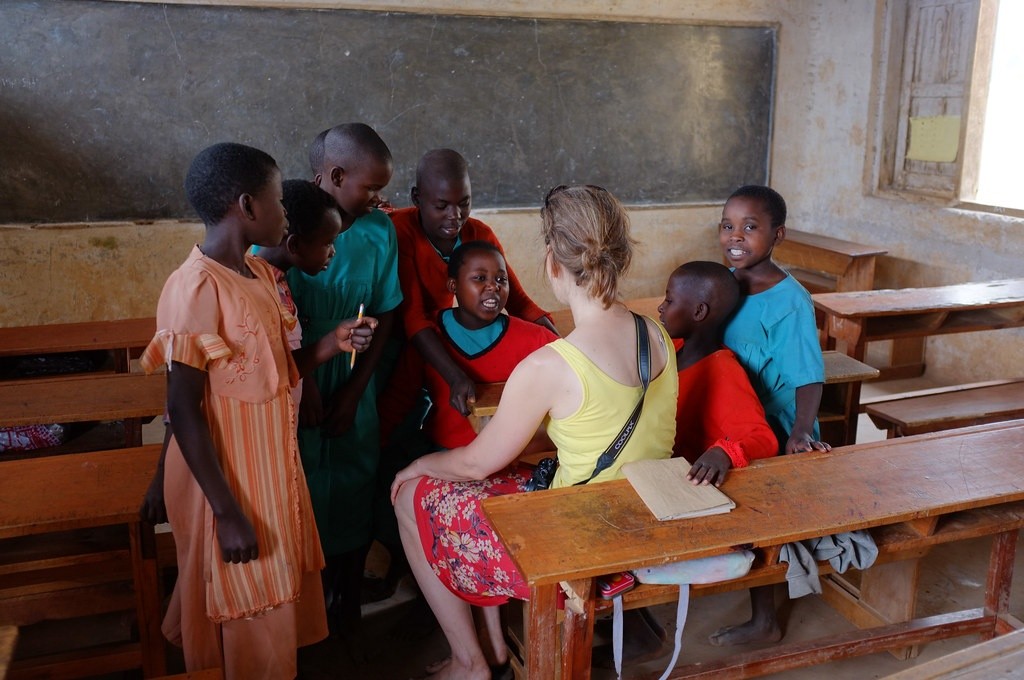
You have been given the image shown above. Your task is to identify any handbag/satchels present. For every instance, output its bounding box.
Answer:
[632,550,755,584]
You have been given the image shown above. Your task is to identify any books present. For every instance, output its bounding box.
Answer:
[622,457,736,521]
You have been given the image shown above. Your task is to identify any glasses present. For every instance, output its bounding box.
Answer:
[545,184,607,208]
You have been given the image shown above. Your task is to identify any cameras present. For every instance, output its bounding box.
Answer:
[525,456,557,492]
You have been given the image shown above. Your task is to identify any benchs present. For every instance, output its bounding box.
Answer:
[865,379,1023,440]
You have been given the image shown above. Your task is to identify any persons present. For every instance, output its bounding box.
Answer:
[591,261,779,669]
[134,123,565,680]
[389,185,679,680]
[710,185,831,647]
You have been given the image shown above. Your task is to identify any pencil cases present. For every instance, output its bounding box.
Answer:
[596,572,635,601]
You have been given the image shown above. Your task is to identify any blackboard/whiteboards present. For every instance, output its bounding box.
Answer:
[0,0,781,226]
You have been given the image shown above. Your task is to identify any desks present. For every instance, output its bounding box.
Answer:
[1,226,1022,680]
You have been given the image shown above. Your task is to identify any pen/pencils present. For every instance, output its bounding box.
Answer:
[351,304,364,370]
